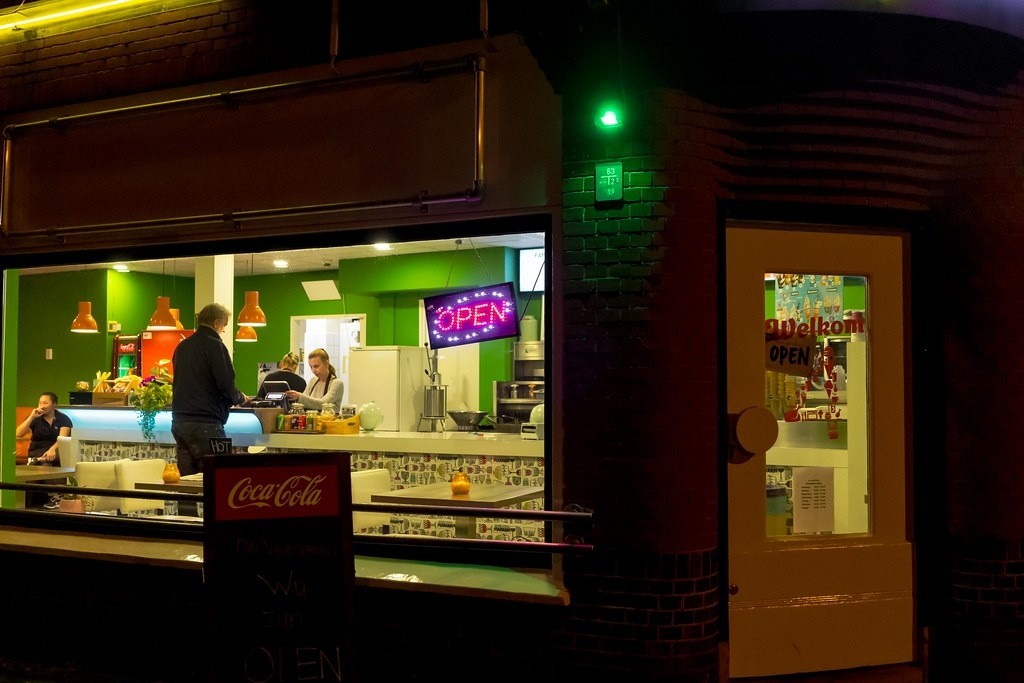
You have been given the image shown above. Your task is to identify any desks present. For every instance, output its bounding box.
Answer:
[15,464,76,508]
[371,480,545,539]
[134,473,203,493]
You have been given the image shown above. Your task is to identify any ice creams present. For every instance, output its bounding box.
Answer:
[777,275,840,323]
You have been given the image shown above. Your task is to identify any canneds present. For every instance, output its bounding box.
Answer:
[278,414,314,430]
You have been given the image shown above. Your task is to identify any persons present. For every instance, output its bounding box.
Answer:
[285,348,344,415]
[257,352,307,415]
[171,303,249,517]
[16,392,73,509]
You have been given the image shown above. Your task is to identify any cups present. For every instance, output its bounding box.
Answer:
[93,379,104,392]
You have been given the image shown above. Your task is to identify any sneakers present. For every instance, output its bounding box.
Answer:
[27,457,41,466]
[43,498,61,509]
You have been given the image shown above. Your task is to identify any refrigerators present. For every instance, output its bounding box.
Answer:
[348,345,430,432]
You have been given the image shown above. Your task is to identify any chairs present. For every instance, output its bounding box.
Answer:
[74,455,203,522]
[351,469,391,534]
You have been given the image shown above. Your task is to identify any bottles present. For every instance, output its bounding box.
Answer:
[277,403,355,430]
[510,384,519,399]
[128,388,134,406]
[452,466,470,494]
[162,455,180,483]
[528,384,537,399]
[519,315,538,342]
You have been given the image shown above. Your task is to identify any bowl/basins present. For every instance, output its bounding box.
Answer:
[447,410,488,431]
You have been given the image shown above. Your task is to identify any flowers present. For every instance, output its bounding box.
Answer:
[130,376,173,450]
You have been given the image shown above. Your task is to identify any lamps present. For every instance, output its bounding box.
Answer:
[71,263,99,333]
[149,258,184,330]
[236,254,267,342]
